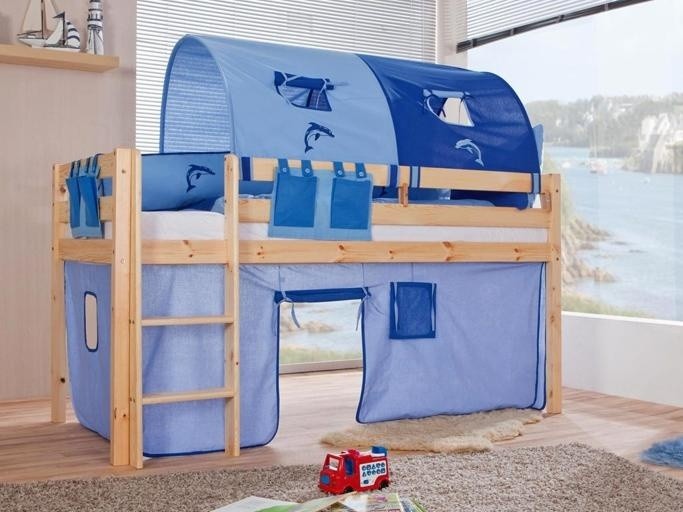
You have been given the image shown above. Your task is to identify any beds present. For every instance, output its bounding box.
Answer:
[47,146,564,472]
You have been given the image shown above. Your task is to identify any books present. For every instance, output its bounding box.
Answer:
[209,490,425,511]
